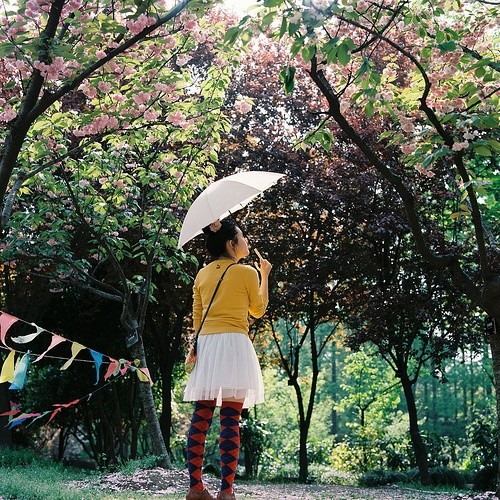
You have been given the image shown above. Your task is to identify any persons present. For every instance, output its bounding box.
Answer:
[181,219,272,500]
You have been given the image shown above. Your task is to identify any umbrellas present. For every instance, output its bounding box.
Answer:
[177,171,288,273]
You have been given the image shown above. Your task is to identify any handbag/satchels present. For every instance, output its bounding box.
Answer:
[185,340,198,373]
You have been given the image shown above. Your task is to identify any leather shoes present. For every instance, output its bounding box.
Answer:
[186,488,217,500]
[217,491,237,500]
